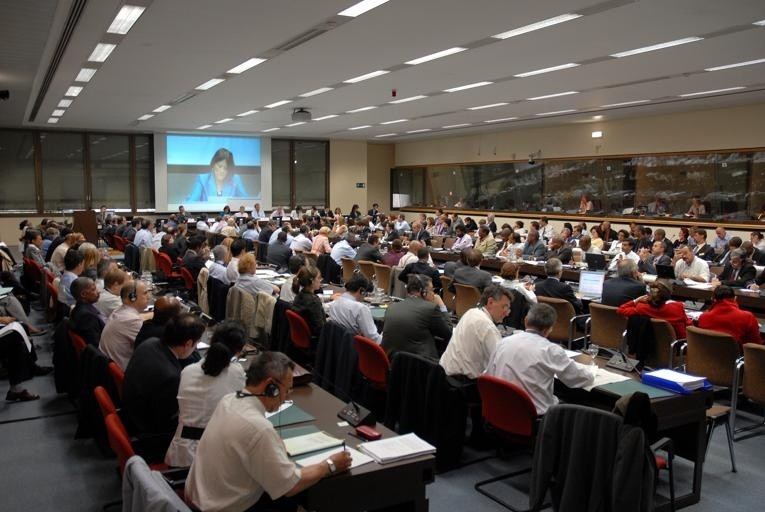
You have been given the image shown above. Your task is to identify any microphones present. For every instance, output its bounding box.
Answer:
[496,320,516,336]
[597,343,640,372]
[673,285,705,312]
[522,264,537,283]
[305,364,376,428]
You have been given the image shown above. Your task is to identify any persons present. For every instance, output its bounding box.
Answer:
[577,193,594,215]
[1,201,764,511]
[686,195,706,218]
[183,146,248,202]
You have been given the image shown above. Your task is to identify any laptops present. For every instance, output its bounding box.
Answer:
[575,270,605,300]
[585,253,608,270]
[656,264,676,280]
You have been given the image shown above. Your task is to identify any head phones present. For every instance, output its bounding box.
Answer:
[414,274,427,297]
[129,279,138,301]
[353,269,369,296]
[236,384,280,398]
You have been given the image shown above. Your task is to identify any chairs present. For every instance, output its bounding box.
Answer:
[0,213,765,511]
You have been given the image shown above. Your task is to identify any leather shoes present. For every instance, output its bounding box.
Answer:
[30,329,47,336]
[35,366,53,376]
[6,389,40,401]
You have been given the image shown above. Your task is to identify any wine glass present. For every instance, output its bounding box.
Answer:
[589,344,599,366]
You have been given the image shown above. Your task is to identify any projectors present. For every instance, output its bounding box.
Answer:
[292,108,311,121]
